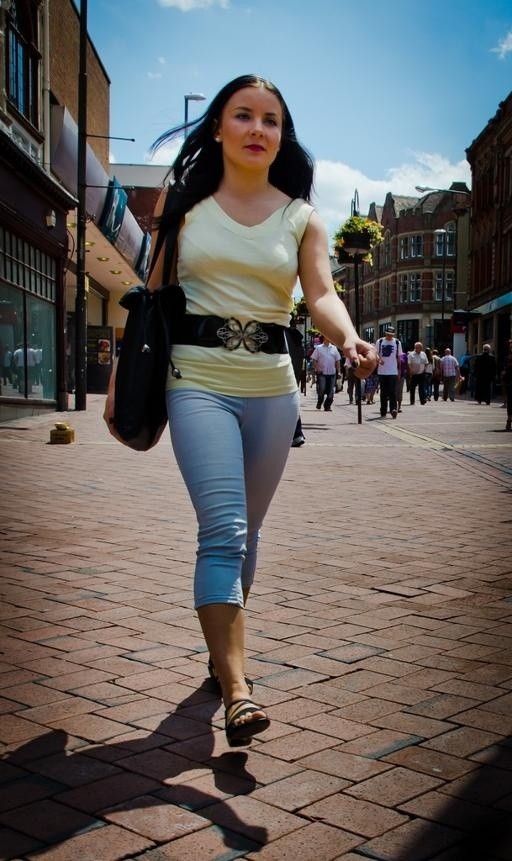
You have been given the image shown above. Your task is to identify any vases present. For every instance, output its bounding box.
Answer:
[308,331,319,338]
[338,292,344,300]
[297,303,308,313]
[337,251,363,264]
[342,232,370,248]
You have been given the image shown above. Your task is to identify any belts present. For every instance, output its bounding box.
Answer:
[172,315,292,354]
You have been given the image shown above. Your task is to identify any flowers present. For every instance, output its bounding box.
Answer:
[334,281,346,293]
[335,215,385,247]
[333,243,374,268]
[305,326,320,336]
[297,299,306,304]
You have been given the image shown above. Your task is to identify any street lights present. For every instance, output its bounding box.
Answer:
[415,186,475,358]
[432,225,448,347]
[184,92,205,176]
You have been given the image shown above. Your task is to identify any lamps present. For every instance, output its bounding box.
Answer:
[45,208,57,229]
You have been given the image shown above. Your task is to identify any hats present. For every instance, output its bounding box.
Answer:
[385,327,395,335]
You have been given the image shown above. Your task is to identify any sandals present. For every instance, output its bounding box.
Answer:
[226,700,270,747]
[207,656,252,698]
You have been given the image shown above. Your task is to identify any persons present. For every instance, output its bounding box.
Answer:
[1,340,44,395]
[101,72,382,749]
[298,322,512,437]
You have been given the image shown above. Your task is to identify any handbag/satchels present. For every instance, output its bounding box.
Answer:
[109,184,186,451]
